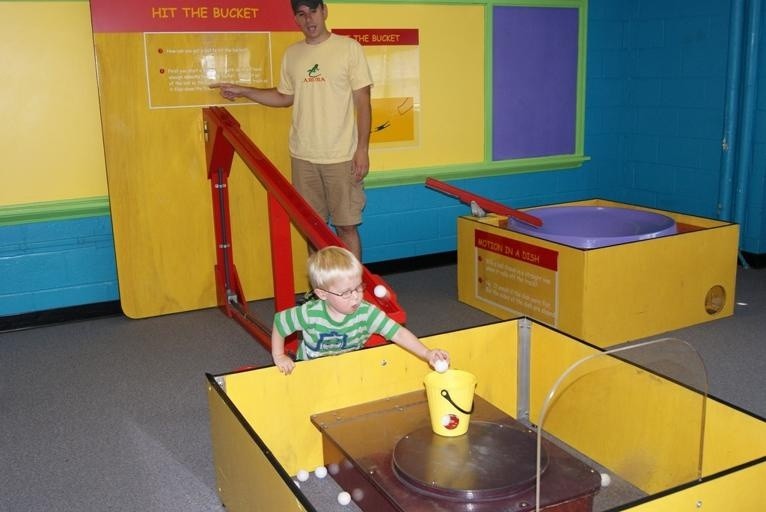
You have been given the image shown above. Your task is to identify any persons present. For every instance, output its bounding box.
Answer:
[265,248,451,382]
[209,1,372,305]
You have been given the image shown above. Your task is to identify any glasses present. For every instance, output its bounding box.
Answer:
[320,282,367,299]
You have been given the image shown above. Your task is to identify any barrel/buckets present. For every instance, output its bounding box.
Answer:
[424,370,479,439]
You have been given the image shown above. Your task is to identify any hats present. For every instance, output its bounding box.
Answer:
[291,0,322,9]
